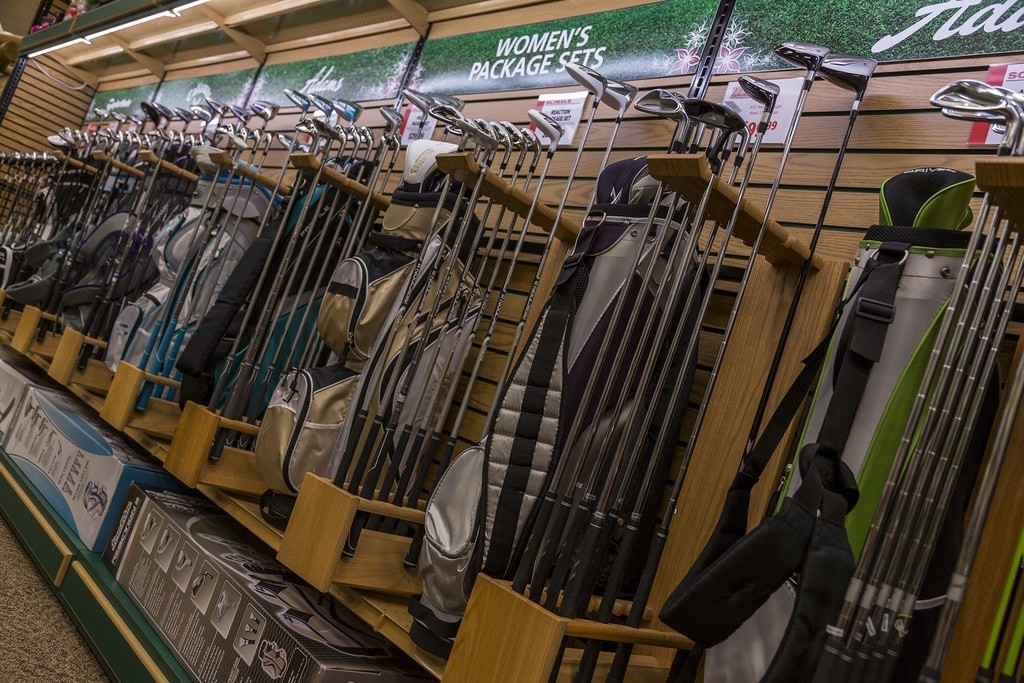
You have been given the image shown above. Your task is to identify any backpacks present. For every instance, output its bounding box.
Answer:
[318,244,417,360]
[149,206,211,282]
[254,364,360,496]
[105,284,171,374]
[405,431,490,658]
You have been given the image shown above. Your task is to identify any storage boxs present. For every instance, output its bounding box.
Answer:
[0,347,406,683]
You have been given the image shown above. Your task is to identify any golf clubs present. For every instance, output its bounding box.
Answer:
[813,78,1023,682]
[489,38,877,683]
[0,86,568,578]
